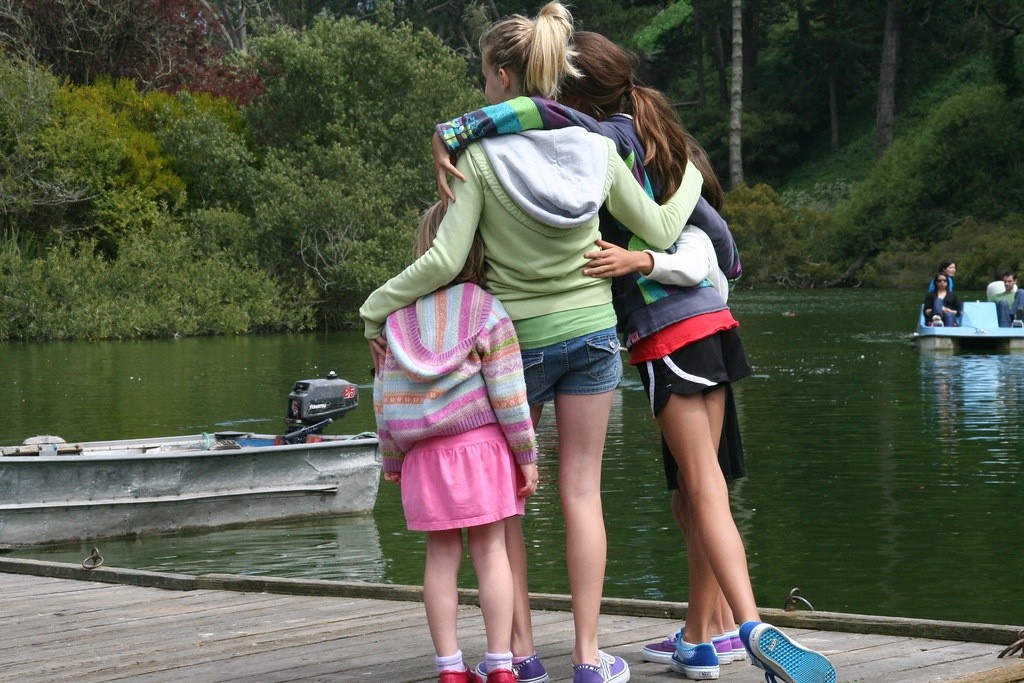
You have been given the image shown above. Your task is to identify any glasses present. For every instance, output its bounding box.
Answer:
[937,280,947,283]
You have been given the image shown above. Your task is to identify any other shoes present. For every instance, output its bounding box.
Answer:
[932,314,941,321]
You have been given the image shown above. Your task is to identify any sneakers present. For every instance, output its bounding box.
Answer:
[641,630,747,664]
[475,655,549,683]
[739,621,837,683]
[438,662,518,683]
[670,627,720,680]
[571,650,630,683]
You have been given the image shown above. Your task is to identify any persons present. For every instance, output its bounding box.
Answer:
[924,260,962,325]
[359,0,836,683]
[991,270,1024,328]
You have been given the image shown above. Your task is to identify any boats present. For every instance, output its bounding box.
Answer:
[911,281,1024,351]
[0,371,384,551]
